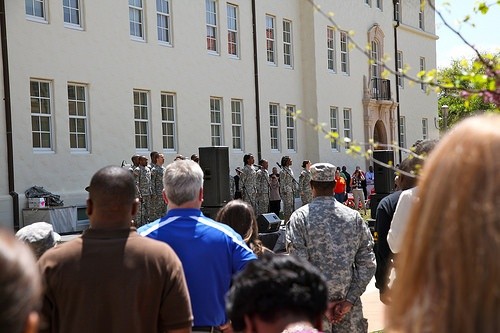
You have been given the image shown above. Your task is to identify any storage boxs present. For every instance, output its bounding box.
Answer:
[28,198,45,208]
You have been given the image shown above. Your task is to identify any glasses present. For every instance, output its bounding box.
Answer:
[84,180,139,193]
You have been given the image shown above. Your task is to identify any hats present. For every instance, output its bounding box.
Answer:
[14,222,62,255]
[306,162,337,185]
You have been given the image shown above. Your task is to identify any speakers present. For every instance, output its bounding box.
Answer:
[373,150,395,193]
[256,212,281,232]
[370,193,390,220]
[198,146,229,206]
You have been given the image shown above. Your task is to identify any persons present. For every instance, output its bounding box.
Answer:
[384,114,500,333]
[137,160,258,333]
[229,153,375,226]
[0,165,194,333]
[215,163,377,333]
[127,152,199,229]
[372,138,438,305]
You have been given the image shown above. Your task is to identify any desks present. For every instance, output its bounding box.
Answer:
[23,206,78,234]
